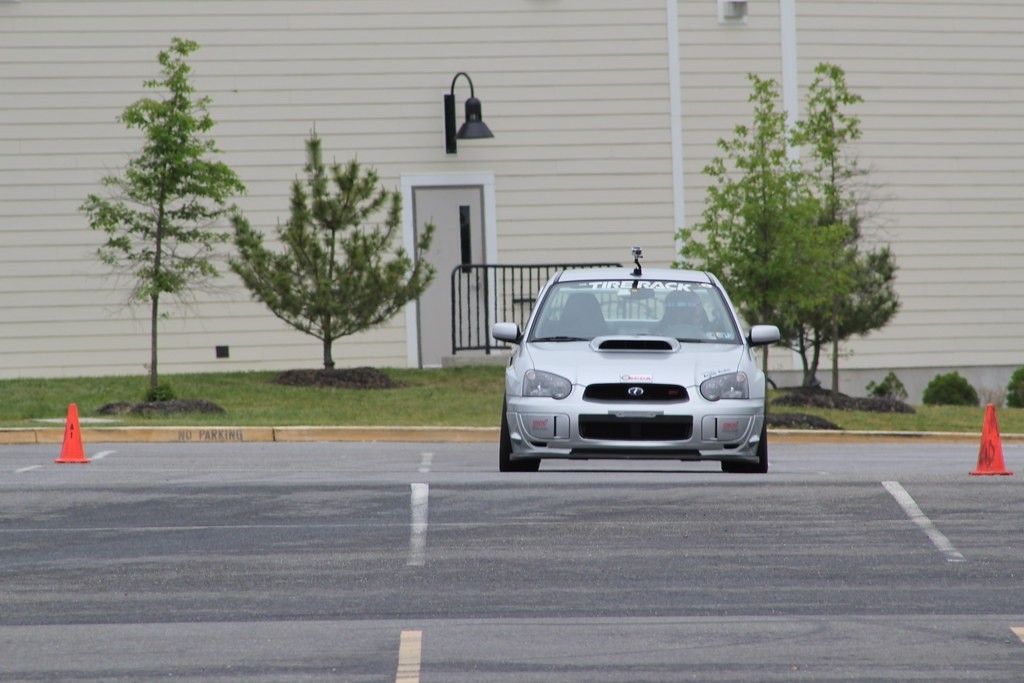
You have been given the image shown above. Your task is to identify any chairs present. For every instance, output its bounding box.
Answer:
[562,293,607,335]
[661,290,710,338]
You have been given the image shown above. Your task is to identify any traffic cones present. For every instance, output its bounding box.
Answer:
[55,401,90,465]
[967,402,1013,477]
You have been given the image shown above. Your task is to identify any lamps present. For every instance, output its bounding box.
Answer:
[444,73,494,153]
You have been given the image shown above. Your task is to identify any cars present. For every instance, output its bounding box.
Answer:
[492,263,781,473]
